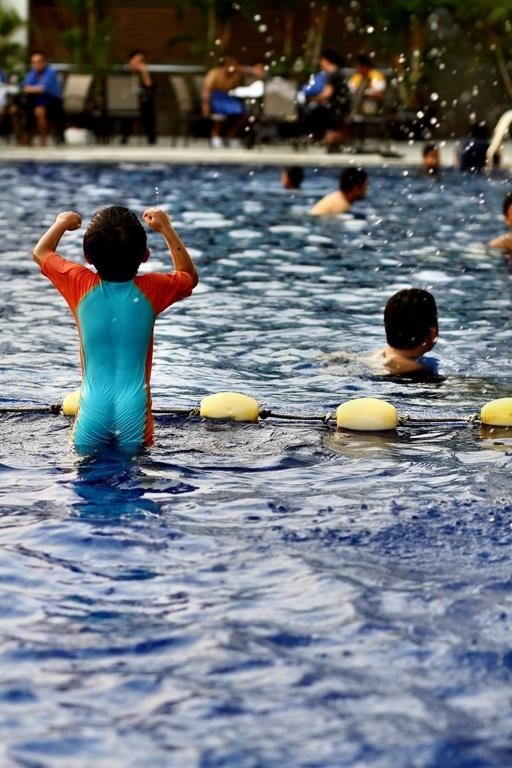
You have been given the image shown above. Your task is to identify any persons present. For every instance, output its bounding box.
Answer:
[201,46,387,148]
[281,166,306,189]
[7,49,64,147]
[0,68,9,120]
[486,190,512,267]
[32,204,198,451]
[356,286,440,381]
[413,140,441,180]
[310,166,370,216]
[117,46,158,143]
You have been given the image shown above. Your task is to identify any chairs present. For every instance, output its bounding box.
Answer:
[53,68,372,152]
